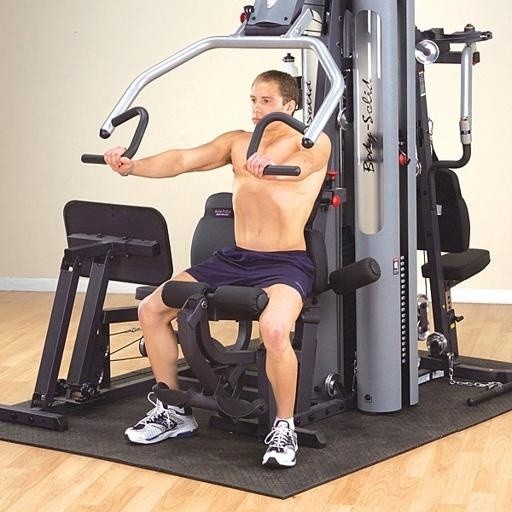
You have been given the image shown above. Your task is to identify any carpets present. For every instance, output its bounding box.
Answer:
[1,329,511,500]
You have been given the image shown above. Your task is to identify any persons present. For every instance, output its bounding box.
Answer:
[102,70,334,468]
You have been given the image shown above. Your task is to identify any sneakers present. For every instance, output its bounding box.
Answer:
[123,389,199,446]
[262,419,299,469]
